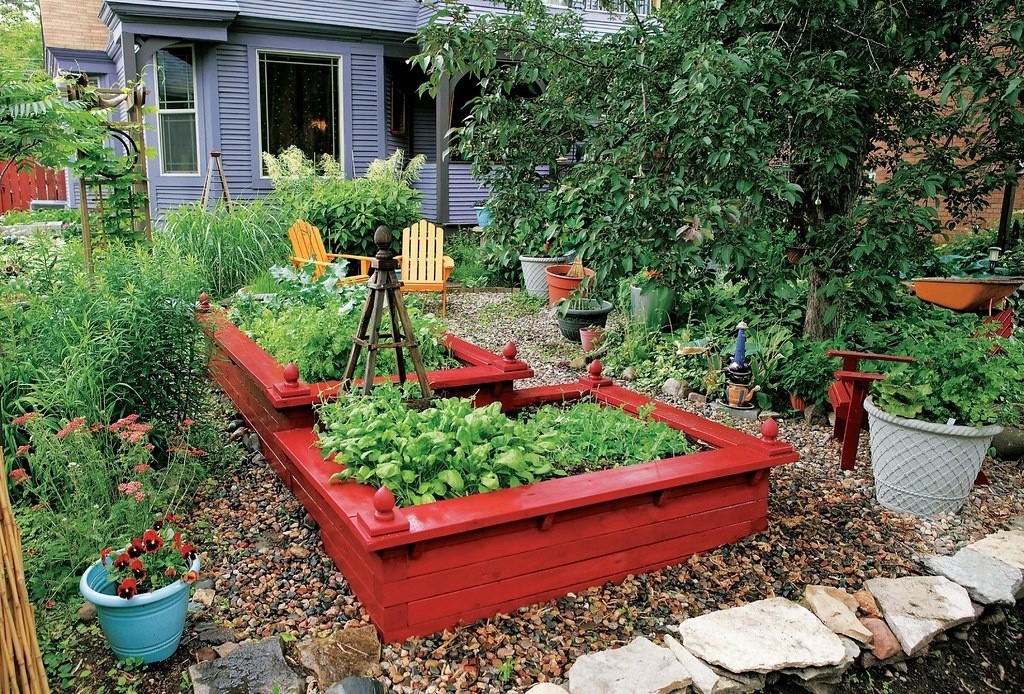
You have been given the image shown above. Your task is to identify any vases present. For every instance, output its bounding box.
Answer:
[630,284,654,324]
[545,264,596,308]
[80,548,200,665]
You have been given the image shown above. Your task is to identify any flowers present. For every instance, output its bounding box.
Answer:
[99,509,202,599]
[630,266,672,297]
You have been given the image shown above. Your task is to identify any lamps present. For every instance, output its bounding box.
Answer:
[988,246,1002,273]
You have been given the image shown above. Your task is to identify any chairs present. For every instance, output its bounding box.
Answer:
[392,220,454,317]
[826,308,1015,472]
[289,220,378,290]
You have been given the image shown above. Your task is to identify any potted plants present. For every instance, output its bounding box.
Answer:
[578,324,606,351]
[198,290,536,455]
[911,254,1024,310]
[862,318,1024,518]
[552,275,615,342]
[272,360,801,647]
[518,233,566,300]
